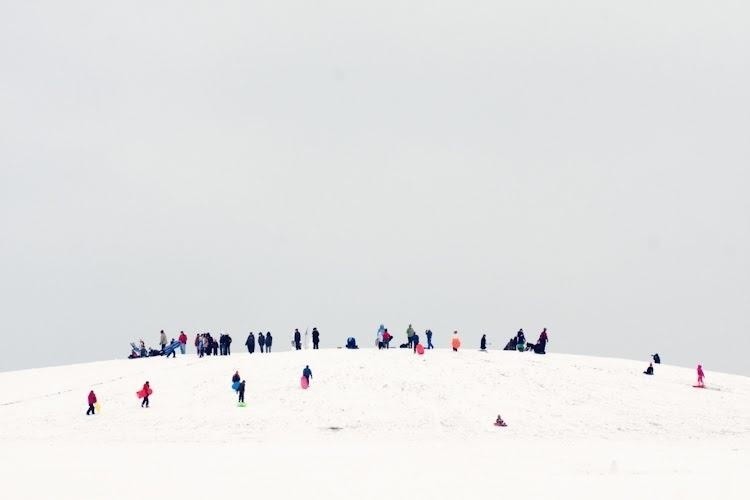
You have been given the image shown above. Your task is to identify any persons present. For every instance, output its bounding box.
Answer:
[481,335,487,350]
[653,354,660,364]
[232,370,240,394]
[239,380,245,403]
[697,365,704,386]
[245,332,255,354]
[415,342,424,354]
[166,338,177,358]
[408,332,419,354]
[496,414,507,426]
[407,326,414,348]
[87,391,97,415]
[346,336,358,349]
[258,332,266,353]
[294,329,302,350]
[383,329,390,349]
[452,330,460,351]
[312,328,320,349]
[266,332,272,353]
[303,365,312,387]
[517,338,525,352]
[130,345,161,358]
[195,332,219,358]
[160,329,167,350]
[644,364,654,375]
[517,329,524,342]
[179,331,187,354]
[425,330,434,350]
[378,332,384,349]
[219,333,231,355]
[141,381,151,408]
[538,328,548,350]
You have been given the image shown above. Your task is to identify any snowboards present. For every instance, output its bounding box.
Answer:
[301,377,307,388]
[137,388,153,398]
[494,420,508,427]
[452,337,461,348]
[239,400,246,407]
[93,403,101,412]
[163,342,181,357]
[417,344,423,353]
